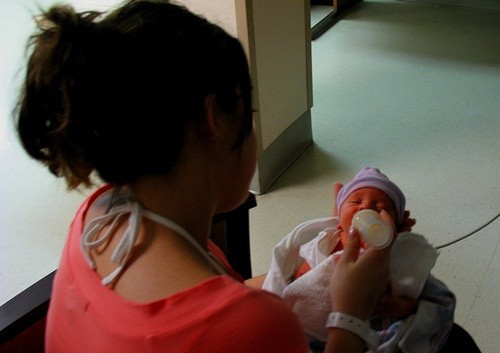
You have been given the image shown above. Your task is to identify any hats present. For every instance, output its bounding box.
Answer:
[337,166,407,221]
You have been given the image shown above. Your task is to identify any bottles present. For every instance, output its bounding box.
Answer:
[351,208,393,249]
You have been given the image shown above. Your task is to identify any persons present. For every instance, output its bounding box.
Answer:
[0,0,482,353]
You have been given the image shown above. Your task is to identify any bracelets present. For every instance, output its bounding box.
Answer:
[325,311,380,351]
